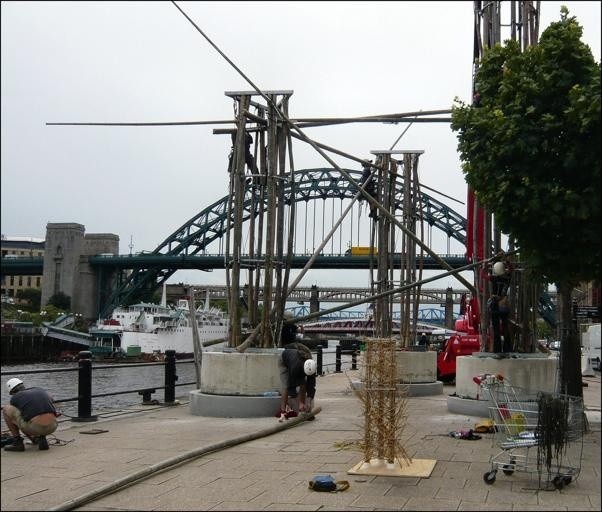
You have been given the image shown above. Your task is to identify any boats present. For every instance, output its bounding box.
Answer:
[93,302,229,360]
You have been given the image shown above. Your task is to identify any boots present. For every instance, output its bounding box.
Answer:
[38,435,49,450]
[4,437,24,451]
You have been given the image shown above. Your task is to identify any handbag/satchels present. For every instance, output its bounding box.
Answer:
[308,476,350,493]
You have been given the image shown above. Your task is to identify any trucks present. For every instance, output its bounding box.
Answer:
[345,247,378,257]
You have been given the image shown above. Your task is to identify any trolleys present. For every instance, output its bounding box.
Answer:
[471,371,586,489]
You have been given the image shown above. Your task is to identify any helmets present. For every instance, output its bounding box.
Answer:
[304,359,317,376]
[6,377,23,394]
[492,262,506,276]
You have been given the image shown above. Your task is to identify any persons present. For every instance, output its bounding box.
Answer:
[360,159,382,221]
[419,332,430,351]
[277,349,318,422]
[3,377,58,452]
[281,315,297,348]
[231,115,260,185]
